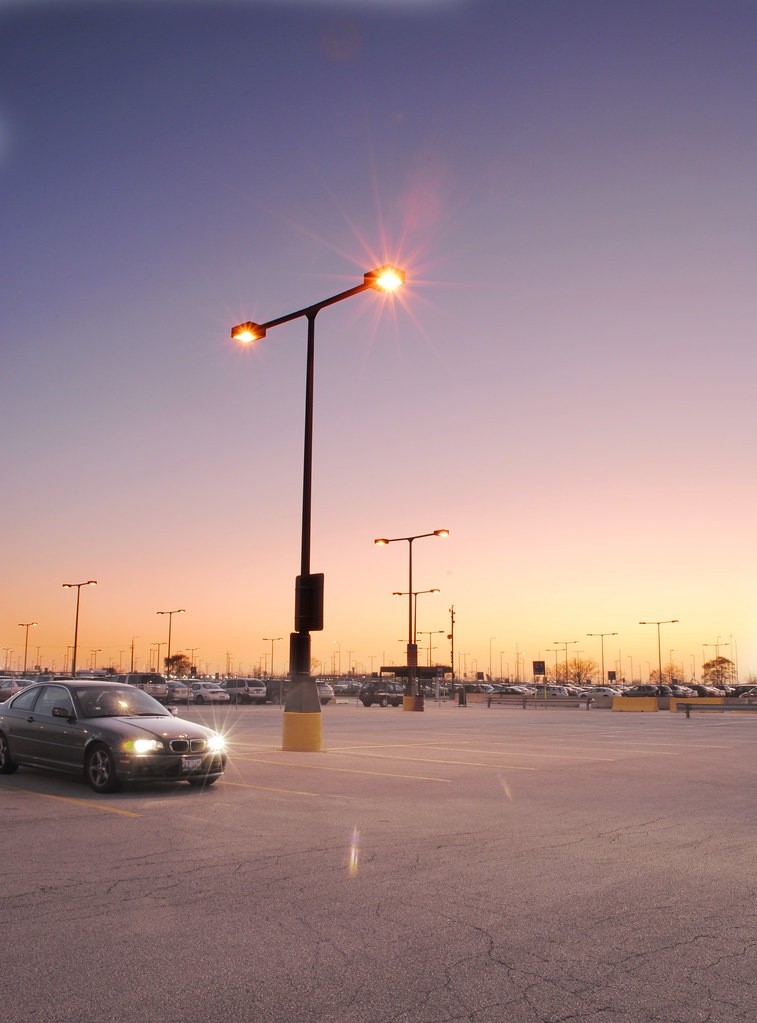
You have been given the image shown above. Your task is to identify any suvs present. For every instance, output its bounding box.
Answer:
[357,681,405,707]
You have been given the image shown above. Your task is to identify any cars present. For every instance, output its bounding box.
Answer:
[0,675,757,704]
[0,680,230,794]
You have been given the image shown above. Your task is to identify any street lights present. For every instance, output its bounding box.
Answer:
[2,635,283,673]
[62,580,98,677]
[319,631,738,685]
[18,622,38,671]
[156,609,186,680]
[262,637,283,676]
[639,620,679,685]
[229,264,406,753]
[391,589,441,643]
[374,529,451,644]
